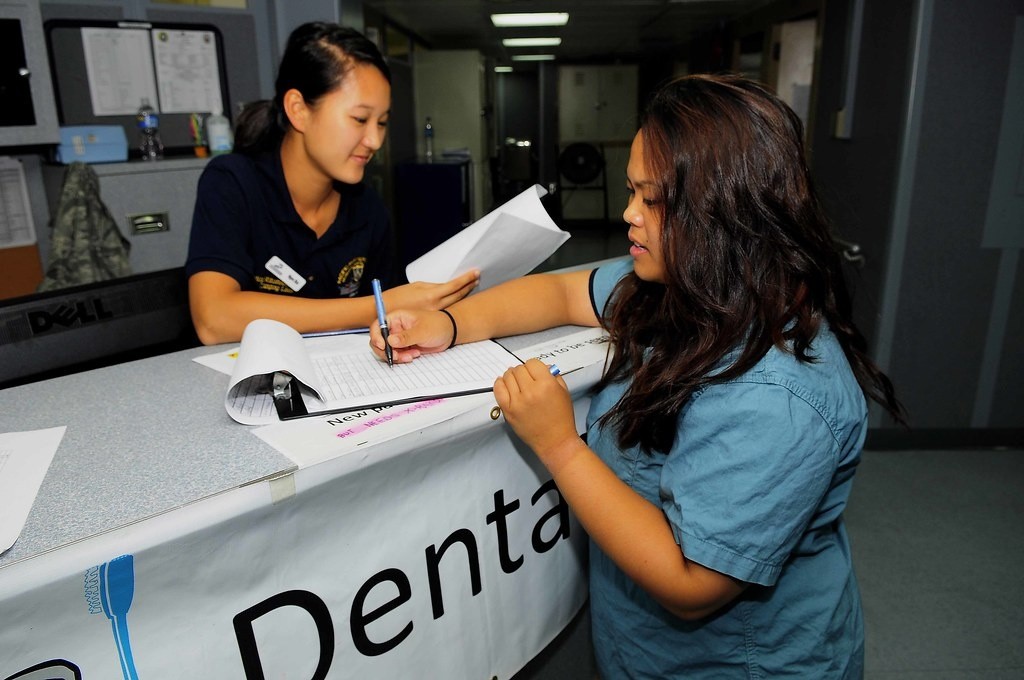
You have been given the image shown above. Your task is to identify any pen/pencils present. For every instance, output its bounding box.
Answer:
[372,278,394,368]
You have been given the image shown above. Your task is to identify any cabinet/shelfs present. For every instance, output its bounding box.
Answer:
[392,152,471,286]
[412,48,498,226]
[557,62,643,234]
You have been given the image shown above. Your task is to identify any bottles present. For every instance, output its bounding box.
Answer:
[206,108,233,157]
[423,117,434,158]
[232,92,246,111]
[137,94,164,161]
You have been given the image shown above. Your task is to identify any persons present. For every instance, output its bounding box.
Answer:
[368,75,895,680]
[183,23,481,347]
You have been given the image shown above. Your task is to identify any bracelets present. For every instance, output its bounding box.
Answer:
[439,309,459,350]
